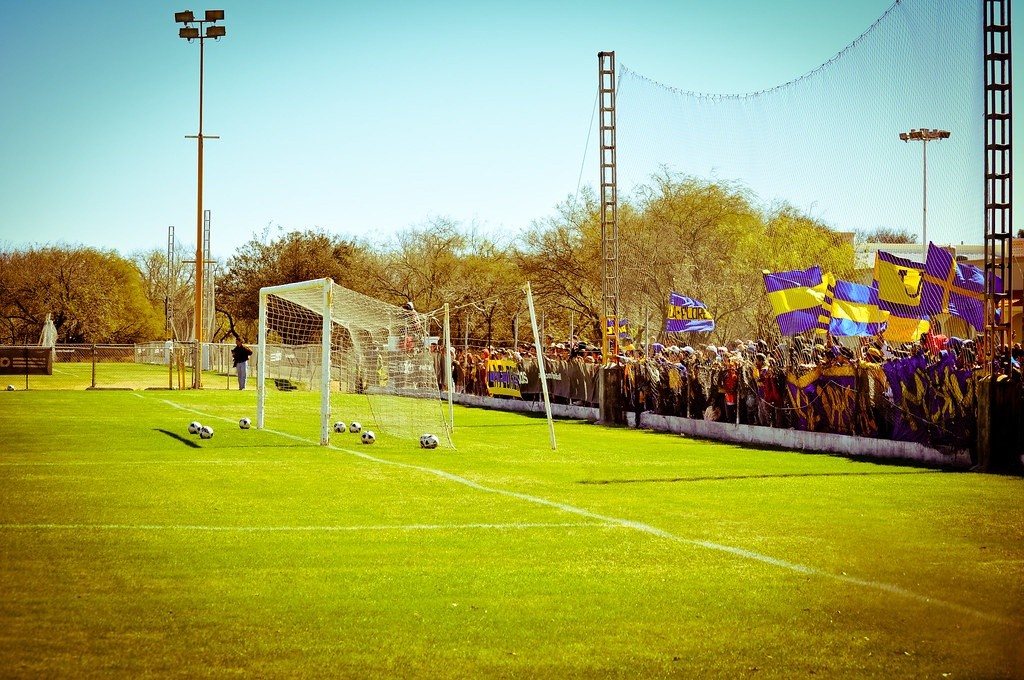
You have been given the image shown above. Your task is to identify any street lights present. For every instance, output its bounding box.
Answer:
[899,127,951,263]
[174,7,227,389]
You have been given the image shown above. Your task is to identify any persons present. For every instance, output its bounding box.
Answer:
[356,333,1024,476]
[402,301,421,333]
[230,337,254,390]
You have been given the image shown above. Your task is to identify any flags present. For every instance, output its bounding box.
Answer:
[873,250,932,320]
[764,266,826,337]
[829,280,890,337]
[919,241,1003,332]
[872,314,929,343]
[666,292,715,332]
[606,320,626,338]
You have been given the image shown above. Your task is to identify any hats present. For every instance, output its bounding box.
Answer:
[479,334,979,363]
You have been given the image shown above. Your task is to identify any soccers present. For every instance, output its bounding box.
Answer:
[333,421,346,433]
[360,430,376,444]
[199,425,214,439]
[7,385,14,391]
[420,433,439,449]
[349,422,362,433]
[188,421,203,434]
[239,417,251,429]
